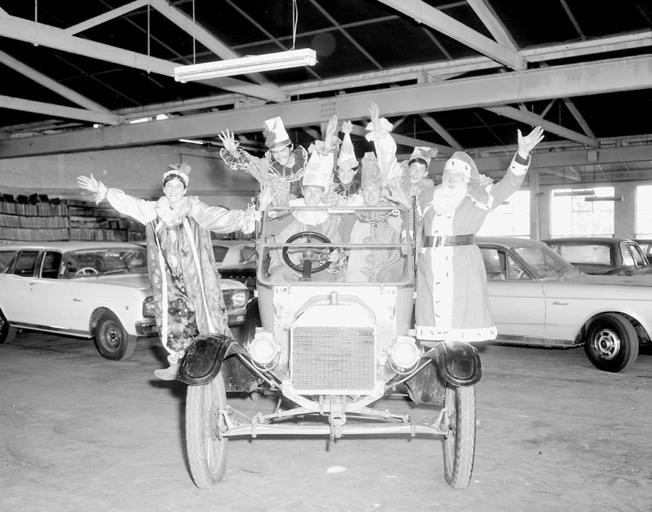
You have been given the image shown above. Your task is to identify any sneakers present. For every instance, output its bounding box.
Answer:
[154,364,182,381]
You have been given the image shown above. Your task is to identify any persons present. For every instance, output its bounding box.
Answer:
[219,102,544,341]
[76,163,262,384]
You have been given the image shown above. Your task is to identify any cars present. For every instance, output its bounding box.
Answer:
[476,236,651,372]
[0,240,249,362]
[129,238,255,267]
[540,236,651,276]
[173,205,482,491]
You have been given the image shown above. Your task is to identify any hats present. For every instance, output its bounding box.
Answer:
[408,146,439,168]
[161,163,191,187]
[262,117,383,191]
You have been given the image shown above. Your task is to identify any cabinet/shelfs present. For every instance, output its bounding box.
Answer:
[0,205,129,242]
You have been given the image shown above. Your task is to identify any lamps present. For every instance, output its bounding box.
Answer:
[554,164,624,201]
[174,0,319,84]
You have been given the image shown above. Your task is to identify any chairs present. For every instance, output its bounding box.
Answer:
[15,268,58,278]
[96,254,130,274]
